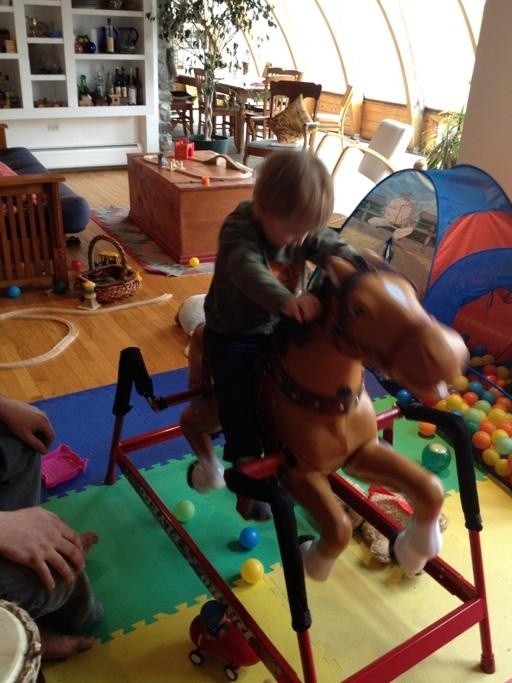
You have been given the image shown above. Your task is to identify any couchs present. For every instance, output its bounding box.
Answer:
[1,119,97,306]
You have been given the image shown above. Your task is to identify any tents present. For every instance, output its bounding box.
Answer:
[337,162,511,362]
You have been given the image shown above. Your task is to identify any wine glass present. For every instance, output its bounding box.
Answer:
[28,16,39,37]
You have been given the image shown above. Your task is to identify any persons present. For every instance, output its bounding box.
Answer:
[0,387,105,664]
[198,147,358,524]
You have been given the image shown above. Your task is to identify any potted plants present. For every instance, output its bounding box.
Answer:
[144,0,280,157]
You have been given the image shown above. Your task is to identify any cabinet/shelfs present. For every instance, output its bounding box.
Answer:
[0,0,164,174]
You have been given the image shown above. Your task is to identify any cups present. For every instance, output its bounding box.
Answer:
[157,152,167,169]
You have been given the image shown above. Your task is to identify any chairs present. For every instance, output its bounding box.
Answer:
[308,118,415,190]
[170,58,360,174]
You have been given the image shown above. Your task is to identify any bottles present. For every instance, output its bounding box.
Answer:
[105,17,114,53]
[1,73,15,108]
[78,64,141,106]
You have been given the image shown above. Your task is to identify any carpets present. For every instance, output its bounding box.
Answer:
[88,202,216,278]
[1,349,511,680]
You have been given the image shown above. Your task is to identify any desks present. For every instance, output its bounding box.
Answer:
[123,146,264,267]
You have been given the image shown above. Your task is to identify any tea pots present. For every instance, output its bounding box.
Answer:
[117,25,139,53]
